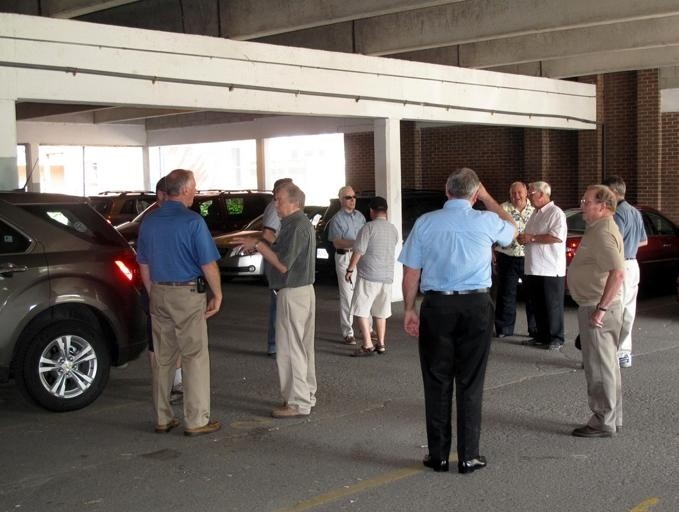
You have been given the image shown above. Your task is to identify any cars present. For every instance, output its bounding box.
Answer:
[1,188,151,412]
[491,205,679,310]
[211,206,329,286]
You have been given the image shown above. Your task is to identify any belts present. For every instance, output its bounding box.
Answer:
[344,249,352,252]
[429,289,491,297]
[157,281,197,286]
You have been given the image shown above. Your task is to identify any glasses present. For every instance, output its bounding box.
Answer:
[342,195,357,199]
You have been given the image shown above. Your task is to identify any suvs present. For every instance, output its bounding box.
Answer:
[114,189,275,250]
[67,190,156,237]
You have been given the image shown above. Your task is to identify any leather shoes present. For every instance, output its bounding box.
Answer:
[271,401,313,417]
[184,422,220,436]
[424,455,449,472]
[156,418,179,434]
[572,425,622,437]
[522,338,564,350]
[458,456,486,473]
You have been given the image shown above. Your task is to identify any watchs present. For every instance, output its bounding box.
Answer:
[530,235,536,243]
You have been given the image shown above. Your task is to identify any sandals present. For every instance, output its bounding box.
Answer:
[344,331,387,357]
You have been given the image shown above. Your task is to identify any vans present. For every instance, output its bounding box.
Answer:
[315,189,483,284]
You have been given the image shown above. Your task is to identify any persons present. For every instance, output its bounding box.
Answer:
[582,175,649,370]
[488,181,539,339]
[516,181,568,352]
[136,169,224,436]
[227,183,318,419]
[397,168,520,473]
[570,185,624,438]
[345,196,398,358]
[140,178,183,405]
[328,185,379,344]
[263,178,293,359]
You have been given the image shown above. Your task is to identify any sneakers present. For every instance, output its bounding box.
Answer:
[619,354,632,368]
[170,383,182,405]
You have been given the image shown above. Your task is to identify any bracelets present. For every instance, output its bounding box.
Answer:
[254,240,262,251]
[346,268,353,273]
[595,304,608,312]
